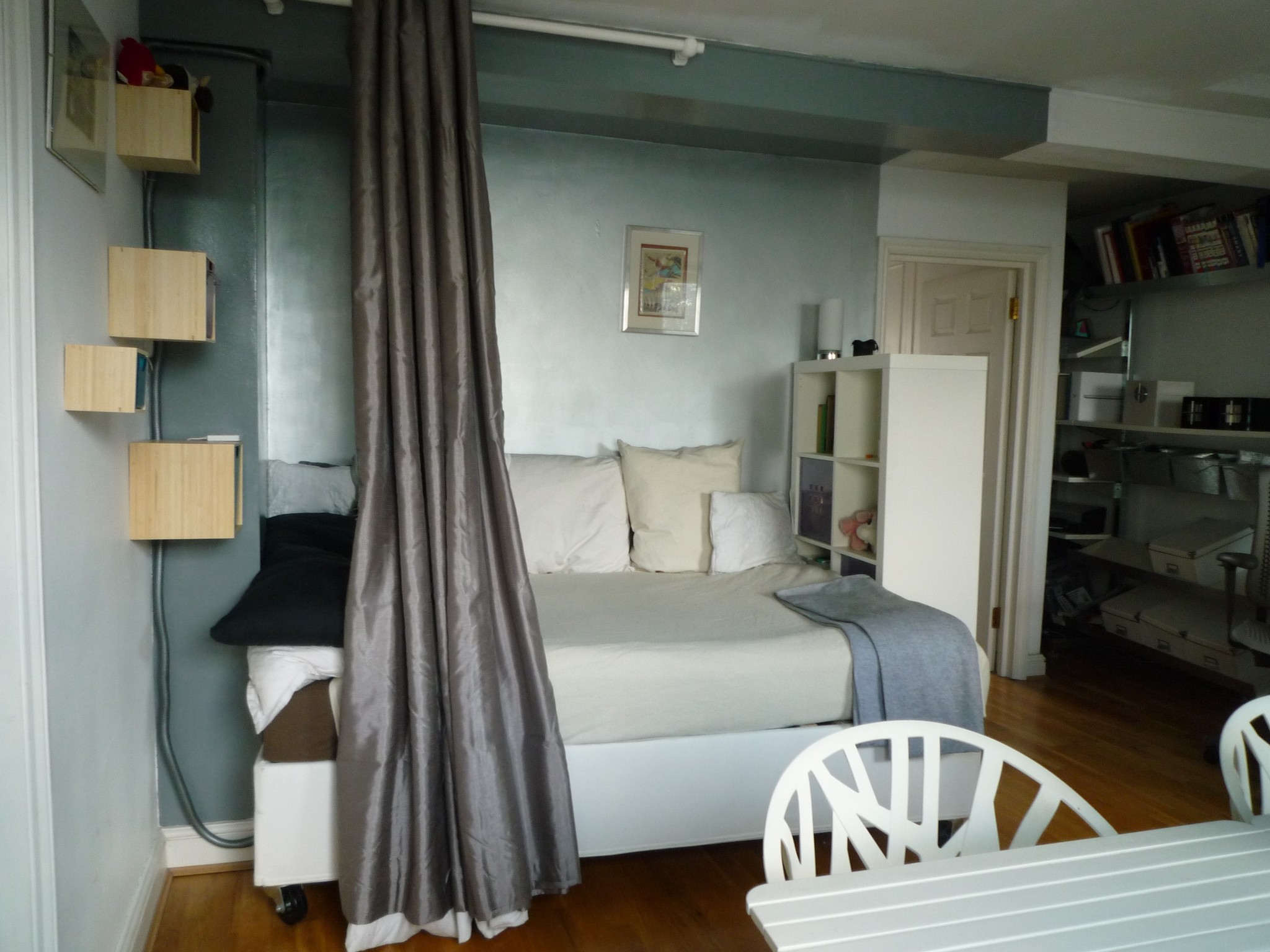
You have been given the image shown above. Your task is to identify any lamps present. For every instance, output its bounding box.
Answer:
[816,296,846,360]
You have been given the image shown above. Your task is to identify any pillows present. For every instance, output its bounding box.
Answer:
[619,441,746,573]
[265,459,358,519]
[501,452,636,574]
[244,643,346,735]
[210,508,360,649]
[708,491,807,573]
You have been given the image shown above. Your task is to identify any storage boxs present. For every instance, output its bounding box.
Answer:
[1151,516,1254,582]
[1101,586,1254,685]
[1068,371,1123,422]
[1124,380,1195,427]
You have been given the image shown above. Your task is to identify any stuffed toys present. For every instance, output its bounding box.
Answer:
[116,38,214,113]
[839,510,877,554]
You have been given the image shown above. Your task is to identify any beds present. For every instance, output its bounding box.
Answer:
[253,569,994,919]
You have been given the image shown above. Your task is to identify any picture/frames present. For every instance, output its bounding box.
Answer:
[621,225,705,336]
[44,2,112,196]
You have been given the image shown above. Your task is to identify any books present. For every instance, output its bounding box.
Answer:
[1092,196,1270,284]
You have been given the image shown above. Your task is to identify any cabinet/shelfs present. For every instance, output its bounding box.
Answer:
[787,354,989,632]
[65,86,244,543]
[1049,422,1270,692]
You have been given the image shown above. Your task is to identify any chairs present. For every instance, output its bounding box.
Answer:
[764,717,1121,884]
[1218,694,1270,821]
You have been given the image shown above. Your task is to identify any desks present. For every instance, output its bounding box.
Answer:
[745,811,1270,952]
